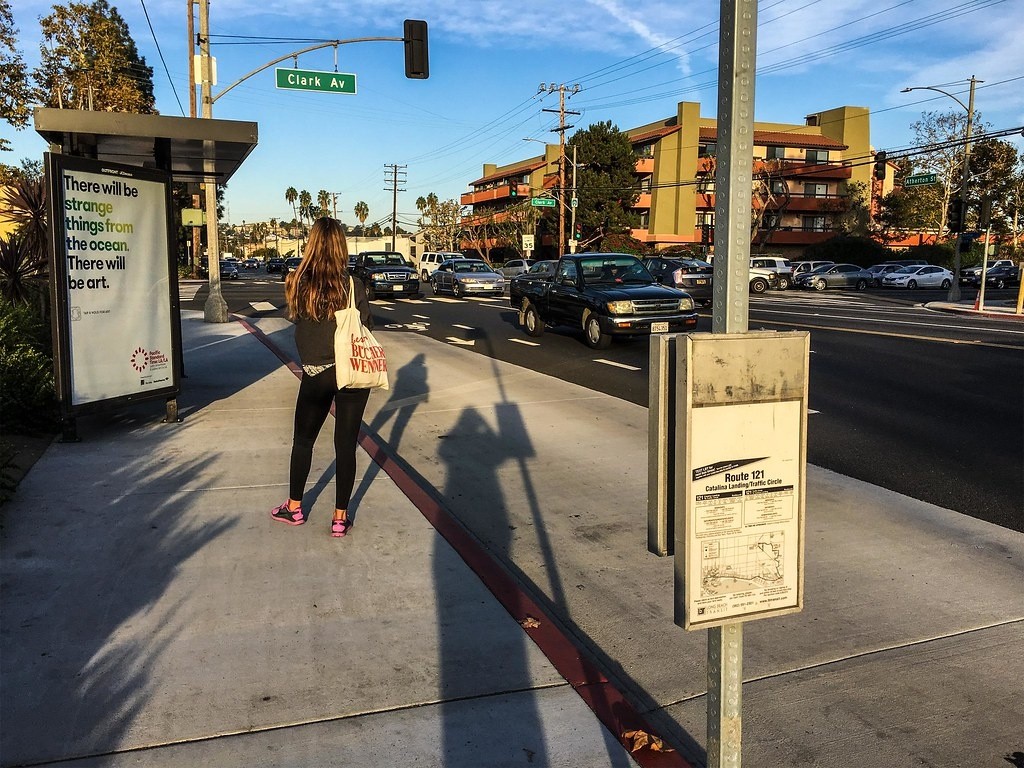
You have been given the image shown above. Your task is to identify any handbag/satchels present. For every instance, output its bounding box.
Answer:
[334,276,389,392]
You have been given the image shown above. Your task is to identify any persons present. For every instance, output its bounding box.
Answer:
[271,217,373,536]
[600,265,620,280]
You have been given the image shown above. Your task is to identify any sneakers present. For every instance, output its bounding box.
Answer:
[271,499,305,525]
[331,518,352,537]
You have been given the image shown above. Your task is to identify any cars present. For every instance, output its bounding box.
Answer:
[959,260,1022,290]
[515,260,593,282]
[429,259,506,298]
[496,259,539,280]
[618,252,714,308]
[281,257,303,282]
[220,257,285,280]
[348,255,359,271]
[706,254,953,291]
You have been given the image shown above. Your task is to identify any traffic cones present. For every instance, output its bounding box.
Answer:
[973,290,981,310]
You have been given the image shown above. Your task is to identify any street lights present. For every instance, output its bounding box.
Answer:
[900,87,973,301]
[522,138,577,254]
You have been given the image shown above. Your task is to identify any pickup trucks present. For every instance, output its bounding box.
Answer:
[511,253,699,350]
[347,251,420,301]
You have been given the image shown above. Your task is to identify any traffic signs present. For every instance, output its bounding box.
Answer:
[905,174,936,187]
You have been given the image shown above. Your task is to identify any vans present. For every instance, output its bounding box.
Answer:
[419,251,471,284]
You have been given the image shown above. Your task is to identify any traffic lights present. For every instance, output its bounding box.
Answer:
[509,178,517,198]
[575,222,582,240]
[947,199,962,232]
[874,151,886,180]
